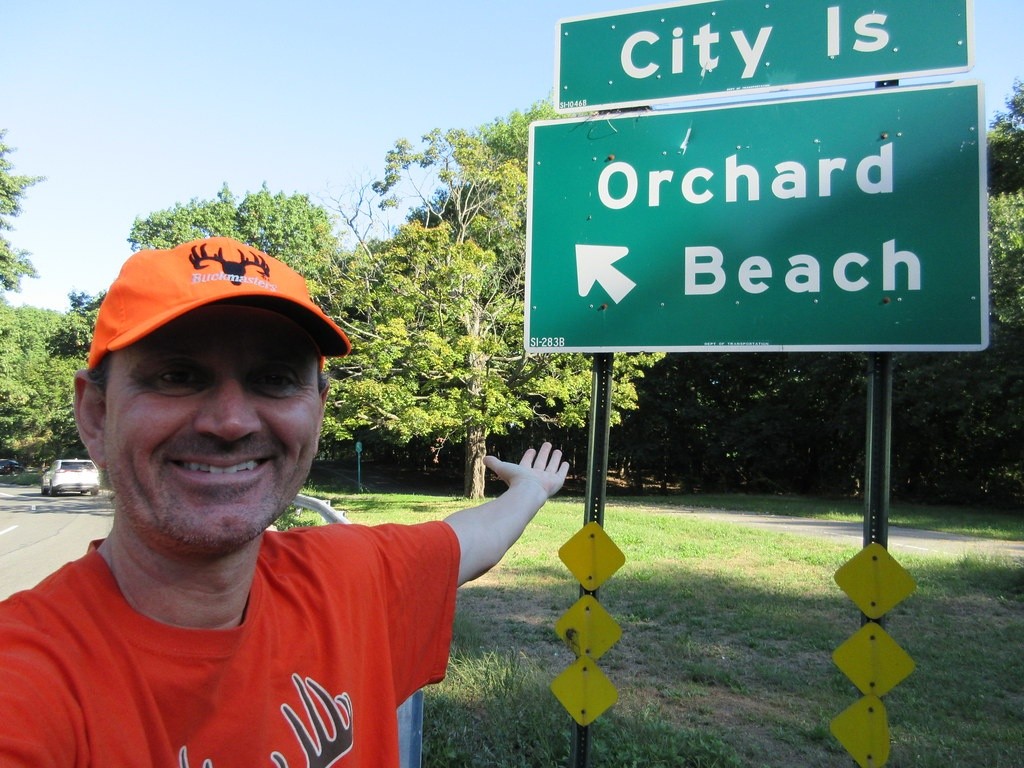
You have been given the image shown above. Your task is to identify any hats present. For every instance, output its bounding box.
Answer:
[87,234,352,379]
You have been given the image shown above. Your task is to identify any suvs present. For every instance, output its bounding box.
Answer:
[41,457,100,497]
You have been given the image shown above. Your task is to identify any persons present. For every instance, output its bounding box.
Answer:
[1,236,575,768]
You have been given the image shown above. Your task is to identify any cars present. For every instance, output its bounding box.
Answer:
[0,459,25,474]
